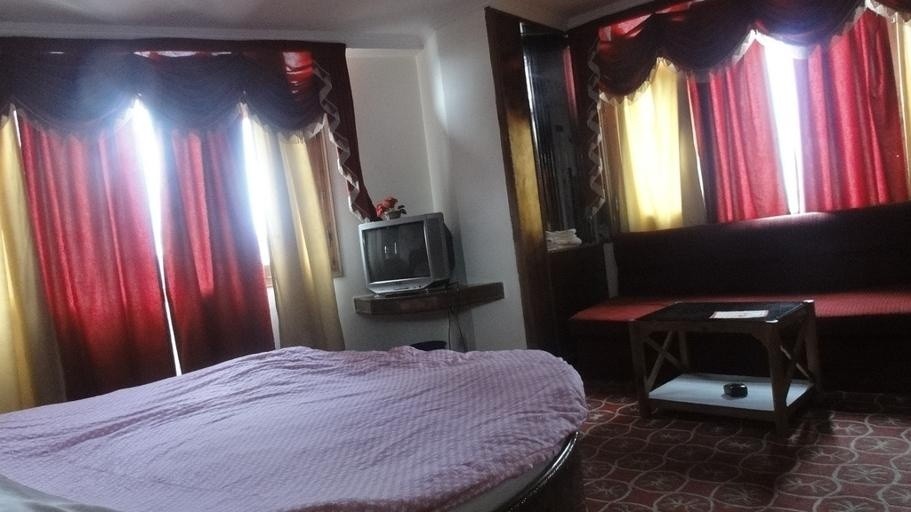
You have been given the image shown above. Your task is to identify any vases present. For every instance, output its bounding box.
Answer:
[388,211,401,220]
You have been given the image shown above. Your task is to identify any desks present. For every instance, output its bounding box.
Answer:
[534,242,610,378]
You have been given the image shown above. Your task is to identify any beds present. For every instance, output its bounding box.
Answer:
[0,345,590,512]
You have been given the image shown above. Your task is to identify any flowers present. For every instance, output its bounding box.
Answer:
[376,197,407,217]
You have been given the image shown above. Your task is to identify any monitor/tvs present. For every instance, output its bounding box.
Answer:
[359,213,455,294]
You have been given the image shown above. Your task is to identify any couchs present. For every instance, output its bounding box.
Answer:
[574,201,911,393]
